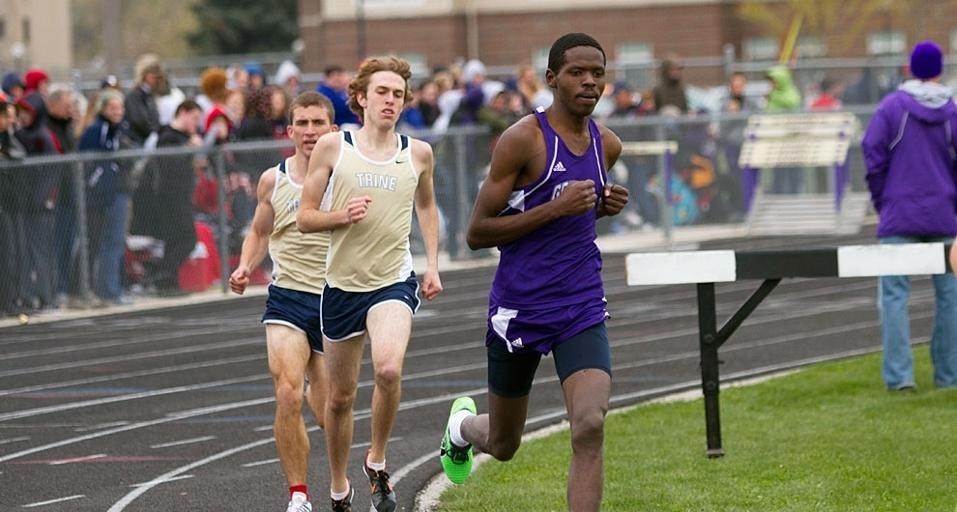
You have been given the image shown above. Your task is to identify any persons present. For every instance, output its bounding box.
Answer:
[188,132,271,287]
[460,59,489,87]
[130,139,198,294]
[296,55,443,512]
[198,66,236,175]
[854,59,887,101]
[1,102,26,317]
[156,98,202,176]
[808,70,846,111]
[13,90,68,310]
[418,76,441,126]
[224,63,250,90]
[24,69,49,96]
[428,63,455,92]
[122,54,163,145]
[273,59,304,95]
[860,42,957,393]
[399,89,424,131]
[684,108,711,149]
[242,61,268,91]
[481,77,507,111]
[651,59,689,116]
[61,90,124,309]
[762,65,803,195]
[516,58,543,100]
[441,86,486,263]
[315,64,362,127]
[720,69,758,213]
[234,83,288,186]
[440,32,628,512]
[505,74,523,112]
[608,80,657,231]
[229,91,339,511]
[1,73,24,99]
[45,87,75,301]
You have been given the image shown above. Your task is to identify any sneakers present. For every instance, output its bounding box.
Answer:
[331,483,354,512]
[284,492,310,512]
[439,394,480,487]
[362,457,397,512]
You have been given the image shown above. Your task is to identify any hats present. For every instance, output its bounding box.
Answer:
[910,43,943,80]
